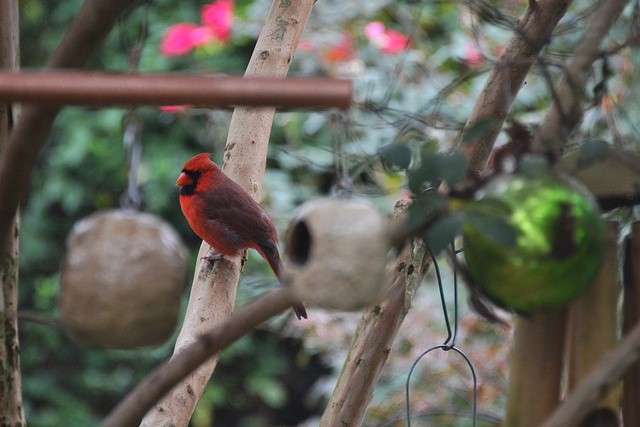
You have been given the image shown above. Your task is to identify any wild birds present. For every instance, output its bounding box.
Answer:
[174,151,309,320]
